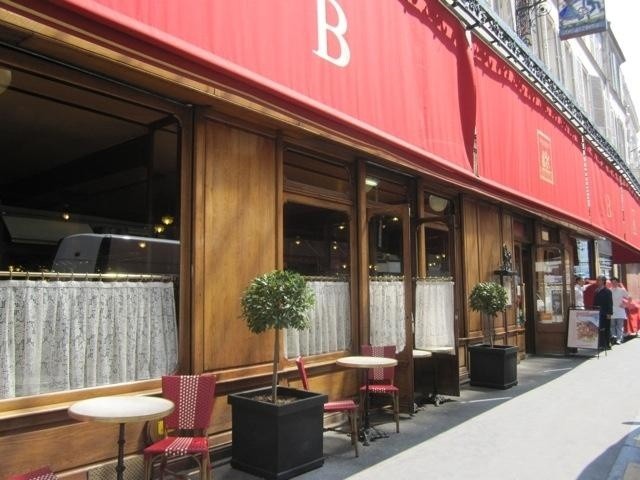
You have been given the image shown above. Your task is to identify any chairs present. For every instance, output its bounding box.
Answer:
[295,356,359,456]
[144,376,216,480]
[8,466,61,480]
[358,345,399,434]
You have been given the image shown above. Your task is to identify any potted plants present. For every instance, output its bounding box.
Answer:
[467,281,519,389]
[227,268,328,480]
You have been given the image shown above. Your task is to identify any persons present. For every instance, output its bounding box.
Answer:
[593,275,614,348]
[574,277,592,309]
[609,277,632,344]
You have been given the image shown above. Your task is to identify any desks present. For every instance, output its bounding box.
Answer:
[68,396,175,480]
[336,356,398,445]
[412,349,431,414]
